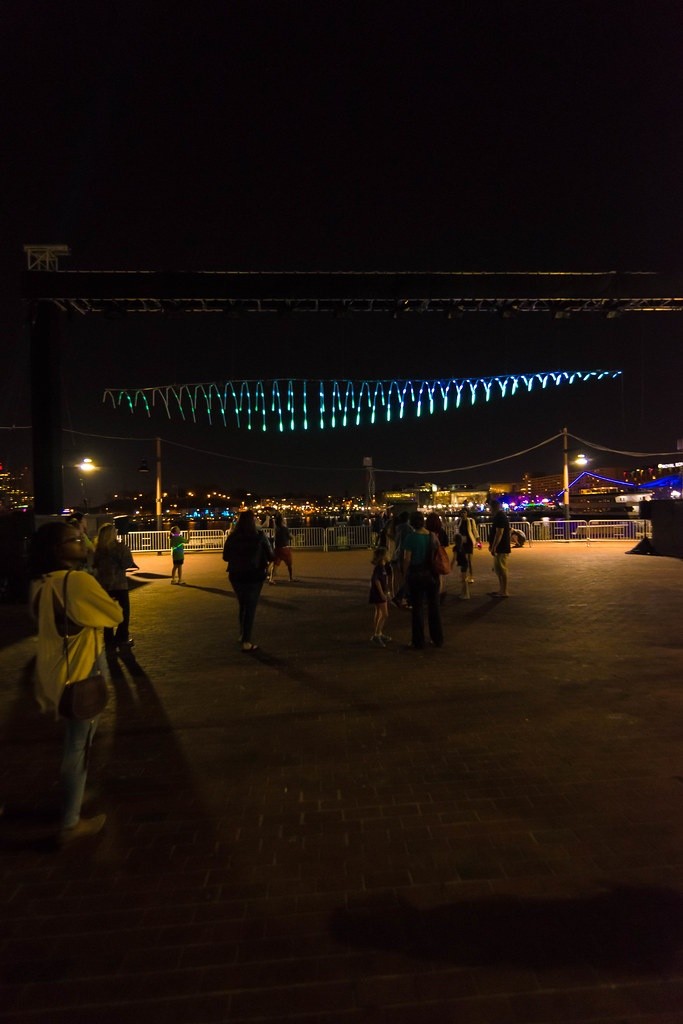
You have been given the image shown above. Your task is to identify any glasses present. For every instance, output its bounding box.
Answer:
[63,535,84,544]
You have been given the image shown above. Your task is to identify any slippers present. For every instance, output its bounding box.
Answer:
[241,642,258,652]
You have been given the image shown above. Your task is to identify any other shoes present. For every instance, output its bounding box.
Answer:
[370,635,392,648]
[468,578,474,583]
[492,592,509,598]
[269,581,276,586]
[288,577,300,582]
[59,813,106,843]
[171,580,186,585]
[430,640,442,648]
[120,639,134,650]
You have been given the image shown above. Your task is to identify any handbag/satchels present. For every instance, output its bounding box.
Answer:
[430,532,450,575]
[58,674,109,722]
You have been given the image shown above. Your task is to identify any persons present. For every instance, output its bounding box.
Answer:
[403,512,444,649]
[370,504,449,608]
[64,514,100,580]
[450,533,471,601]
[269,515,300,585]
[169,526,191,584]
[487,500,511,597]
[509,527,526,548]
[94,522,134,646]
[368,548,392,648]
[454,507,481,583]
[222,510,275,651]
[223,512,273,582]
[27,519,124,848]
